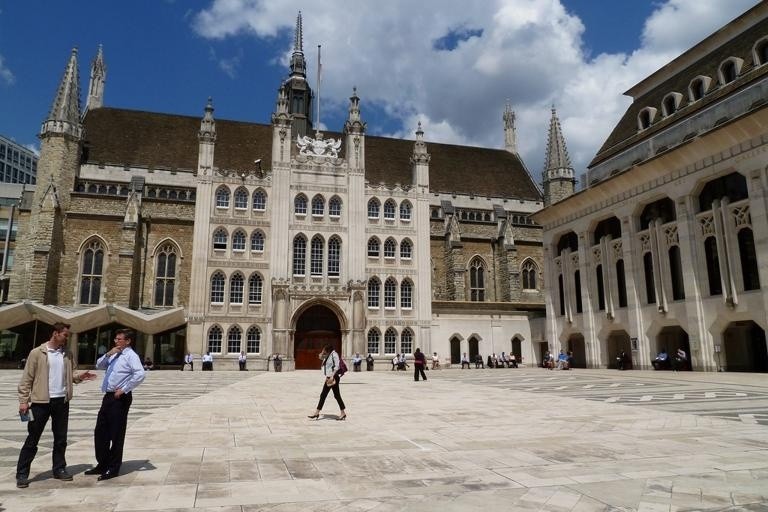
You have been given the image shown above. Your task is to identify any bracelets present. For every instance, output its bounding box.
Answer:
[105,353,111,358]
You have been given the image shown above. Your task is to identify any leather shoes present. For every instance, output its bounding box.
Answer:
[85,464,119,481]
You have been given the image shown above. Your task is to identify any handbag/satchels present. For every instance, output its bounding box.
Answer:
[336,358,347,377]
[326,378,336,387]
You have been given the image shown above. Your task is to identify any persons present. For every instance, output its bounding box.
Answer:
[490,351,517,368]
[676,348,686,371]
[181,353,193,371]
[432,352,439,369]
[366,354,374,371]
[238,351,246,370]
[85,328,146,481]
[353,352,362,372]
[461,352,471,370]
[475,354,485,369]
[16,322,97,487]
[308,344,347,420]
[392,354,410,371]
[144,357,154,370]
[544,350,570,370]
[651,350,667,370]
[201,351,213,370]
[413,348,427,381]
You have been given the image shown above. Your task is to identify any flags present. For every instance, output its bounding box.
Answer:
[317,49,323,84]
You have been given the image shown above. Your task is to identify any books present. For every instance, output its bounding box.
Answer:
[21,409,34,421]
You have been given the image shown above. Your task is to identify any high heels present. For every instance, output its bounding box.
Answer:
[336,415,346,420]
[308,414,319,420]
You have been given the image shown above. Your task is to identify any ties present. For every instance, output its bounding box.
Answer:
[102,353,122,393]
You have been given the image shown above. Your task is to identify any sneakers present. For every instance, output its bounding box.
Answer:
[17,478,28,488]
[54,468,72,481]
